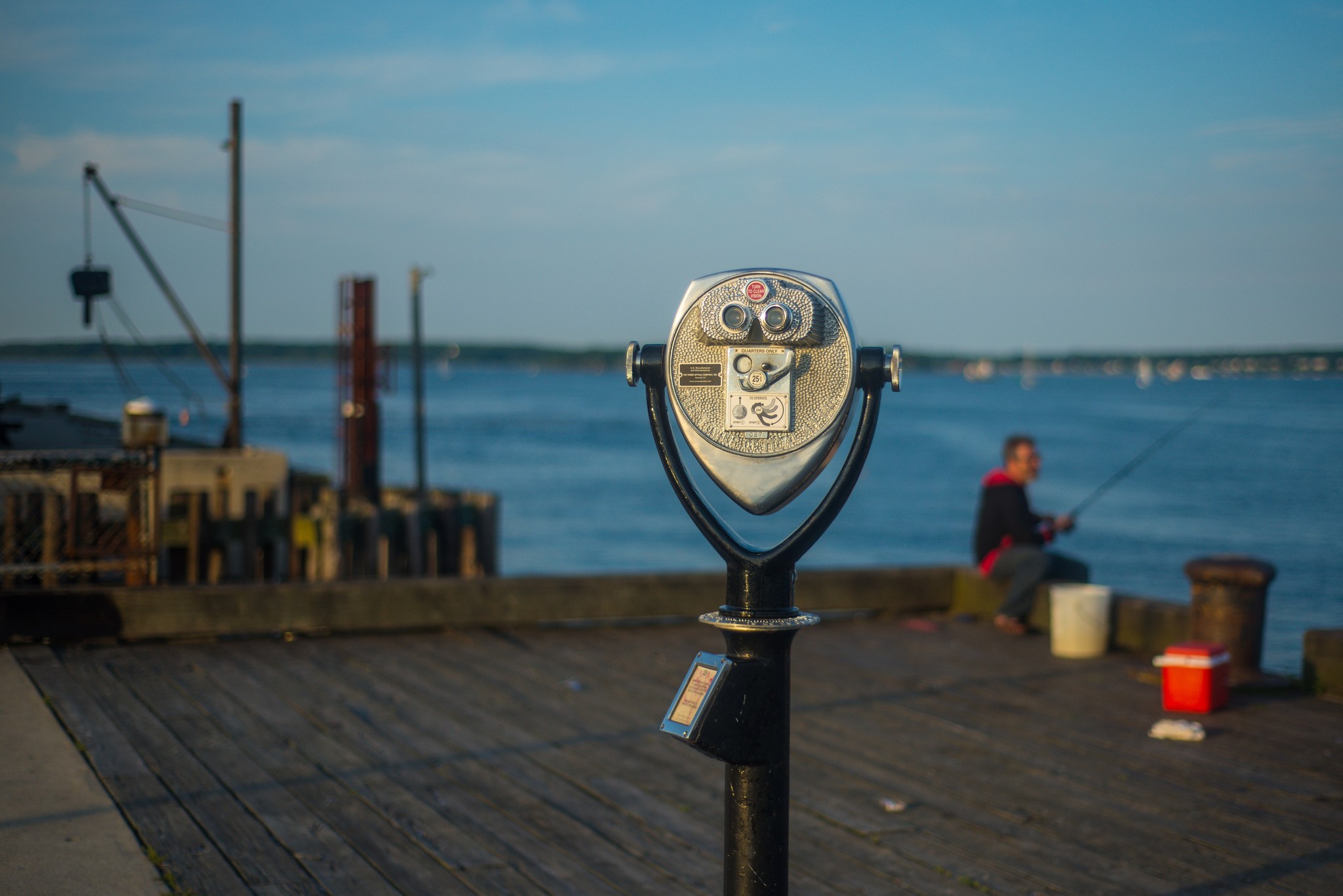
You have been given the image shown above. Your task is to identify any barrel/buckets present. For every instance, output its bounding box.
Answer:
[1048,583,1112,658]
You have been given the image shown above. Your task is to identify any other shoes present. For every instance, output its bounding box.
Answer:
[993,614,1030,637]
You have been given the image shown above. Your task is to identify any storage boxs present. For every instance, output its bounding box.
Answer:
[1153,640,1230,714]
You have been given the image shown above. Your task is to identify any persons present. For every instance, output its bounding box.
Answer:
[967,433,1089,636]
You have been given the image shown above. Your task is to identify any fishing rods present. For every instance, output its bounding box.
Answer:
[1061,389,1234,535]
